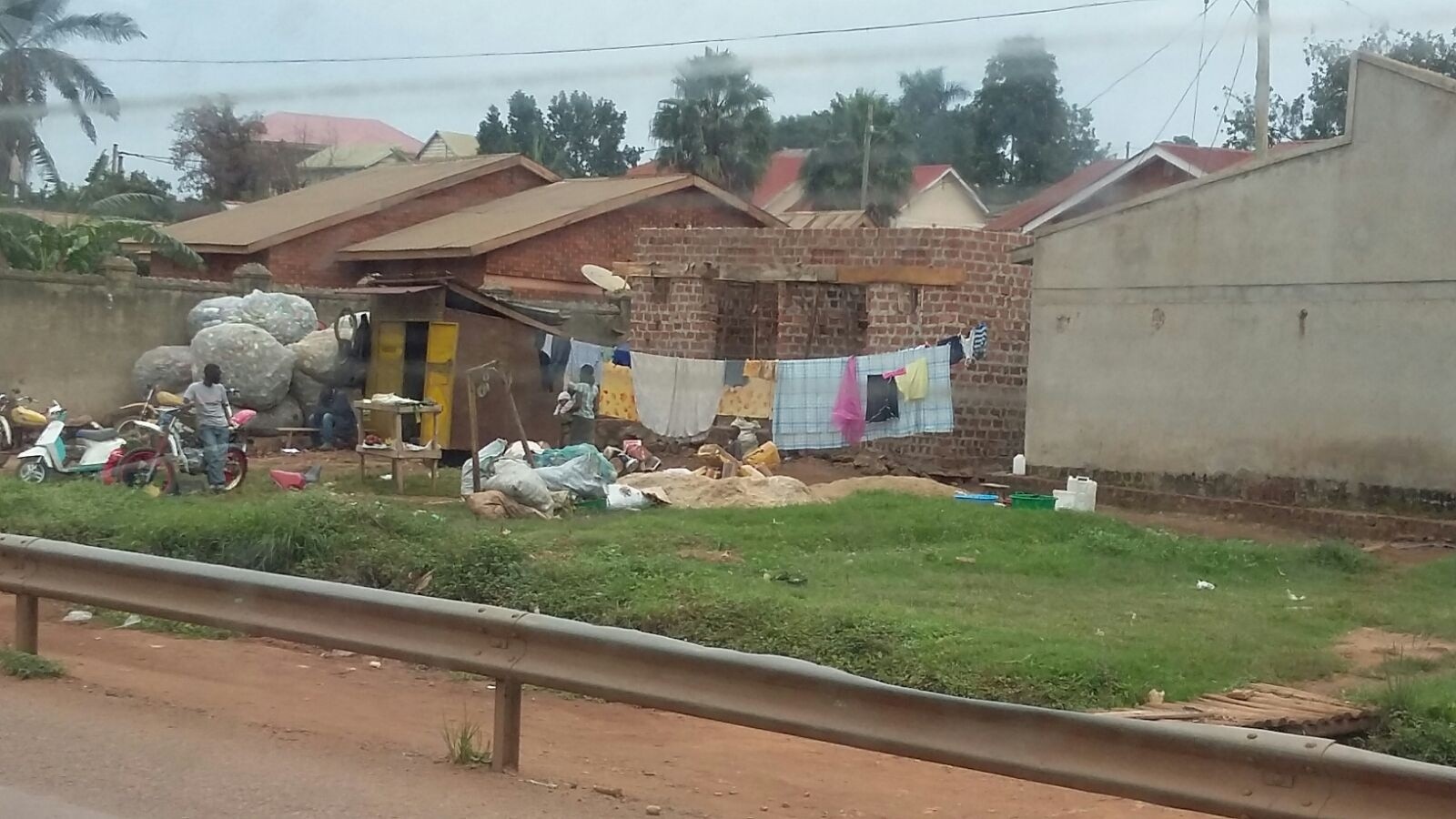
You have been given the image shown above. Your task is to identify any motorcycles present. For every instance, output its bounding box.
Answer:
[1,389,101,453]
[113,382,255,448]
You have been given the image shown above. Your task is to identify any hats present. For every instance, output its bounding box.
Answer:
[558,391,571,402]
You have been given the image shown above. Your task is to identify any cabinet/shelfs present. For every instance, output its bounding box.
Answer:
[354,400,445,496]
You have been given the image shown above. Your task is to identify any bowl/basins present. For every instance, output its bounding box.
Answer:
[954,494,998,505]
[1010,491,1059,510]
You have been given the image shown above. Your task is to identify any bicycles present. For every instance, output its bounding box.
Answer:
[116,408,247,500]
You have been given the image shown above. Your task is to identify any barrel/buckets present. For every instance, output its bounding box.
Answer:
[1052,490,1076,510]
[1067,475,1098,511]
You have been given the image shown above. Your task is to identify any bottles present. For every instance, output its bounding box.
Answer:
[1012,453,1026,475]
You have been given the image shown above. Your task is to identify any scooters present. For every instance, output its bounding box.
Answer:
[16,399,127,489]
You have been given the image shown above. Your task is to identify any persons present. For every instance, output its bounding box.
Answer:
[553,391,573,422]
[567,363,599,445]
[178,364,237,493]
[309,385,358,452]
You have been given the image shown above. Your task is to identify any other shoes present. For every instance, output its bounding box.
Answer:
[210,486,227,494]
[319,442,335,451]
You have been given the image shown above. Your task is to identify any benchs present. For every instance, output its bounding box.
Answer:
[276,427,319,453]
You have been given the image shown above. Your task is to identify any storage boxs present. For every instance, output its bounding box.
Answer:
[1009,493,1057,510]
[955,494,998,509]
